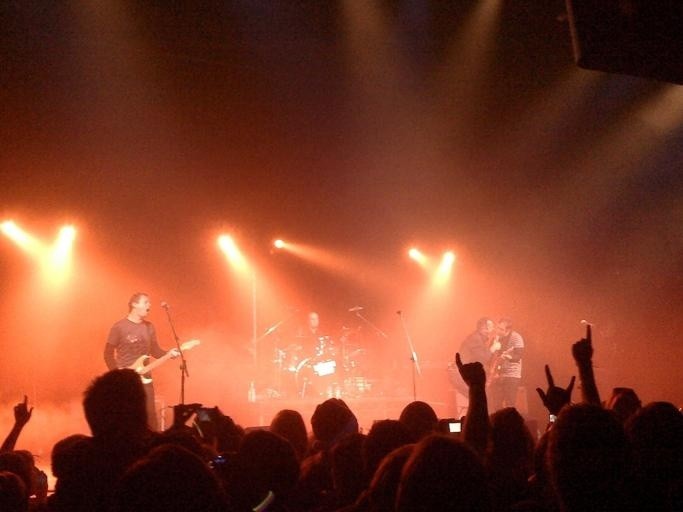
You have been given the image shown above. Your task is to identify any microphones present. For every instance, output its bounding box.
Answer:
[348,306,364,312]
[160,303,170,308]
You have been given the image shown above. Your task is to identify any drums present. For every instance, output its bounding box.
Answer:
[282,358,326,396]
[341,377,371,393]
[307,336,335,360]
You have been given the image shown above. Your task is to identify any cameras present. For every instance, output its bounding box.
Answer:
[446,419,462,433]
[549,414,556,423]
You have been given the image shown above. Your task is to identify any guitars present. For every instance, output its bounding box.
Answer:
[118,339,200,384]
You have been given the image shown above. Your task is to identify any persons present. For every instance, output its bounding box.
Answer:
[488,315,525,407]
[102,293,180,433]
[446,314,501,401]
[297,307,328,356]
[0,321,682,512]
